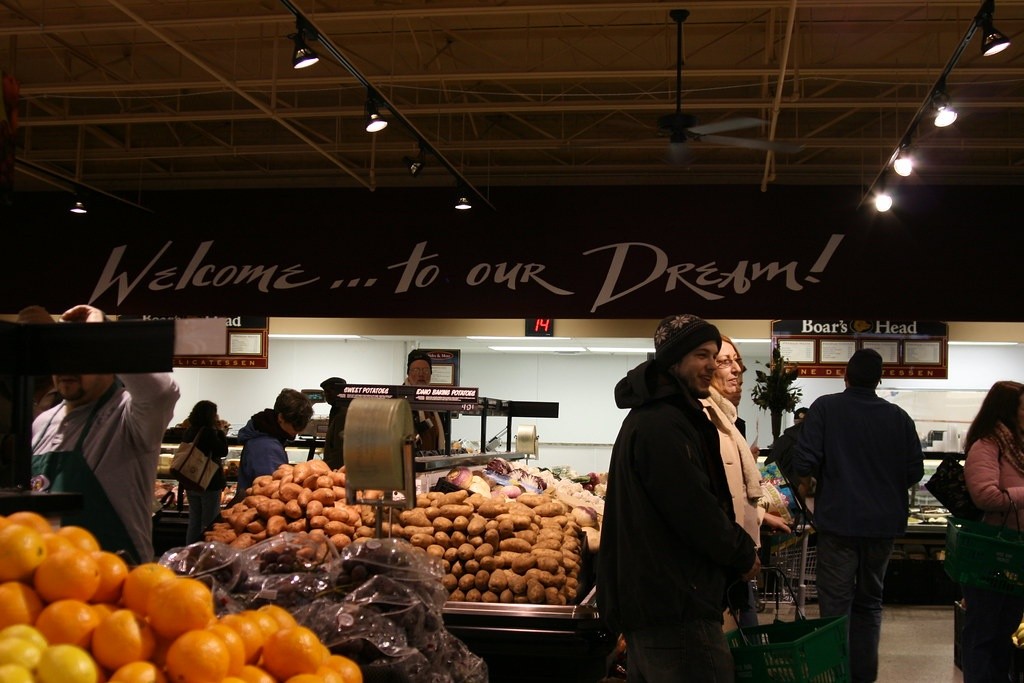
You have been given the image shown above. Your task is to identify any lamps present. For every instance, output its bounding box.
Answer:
[980,21,1013,58]
[933,93,958,129]
[893,144,924,177]
[285,16,319,71]
[453,177,474,210]
[404,146,430,175]
[70,186,86,216]
[362,85,388,132]
[871,174,894,212]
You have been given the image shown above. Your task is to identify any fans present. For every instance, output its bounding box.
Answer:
[617,9,804,157]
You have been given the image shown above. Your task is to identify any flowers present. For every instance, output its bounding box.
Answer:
[752,347,803,411]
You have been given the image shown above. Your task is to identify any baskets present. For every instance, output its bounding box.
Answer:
[724,566,850,683]
[939,487,1024,601]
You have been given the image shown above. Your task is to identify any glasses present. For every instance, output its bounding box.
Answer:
[718,357,740,367]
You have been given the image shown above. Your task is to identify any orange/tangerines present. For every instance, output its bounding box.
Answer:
[0,514,363,683]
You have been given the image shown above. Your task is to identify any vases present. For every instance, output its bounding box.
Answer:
[766,409,783,447]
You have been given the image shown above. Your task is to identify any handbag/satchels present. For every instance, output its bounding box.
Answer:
[924,433,1003,518]
[170,426,222,494]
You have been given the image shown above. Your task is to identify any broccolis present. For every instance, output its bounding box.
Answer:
[508,460,604,513]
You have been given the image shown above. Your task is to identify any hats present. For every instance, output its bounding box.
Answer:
[407,349,433,374]
[320,378,346,390]
[653,313,722,364]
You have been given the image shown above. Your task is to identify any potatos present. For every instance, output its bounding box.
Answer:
[203,458,384,565]
[383,490,585,606]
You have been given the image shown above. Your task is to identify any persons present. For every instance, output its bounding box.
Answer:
[237,389,314,496]
[394,349,444,458]
[764,406,812,540]
[319,377,353,470]
[790,348,925,683]
[596,315,790,683]
[962,382,1024,683]
[30,303,180,567]
[180,400,233,547]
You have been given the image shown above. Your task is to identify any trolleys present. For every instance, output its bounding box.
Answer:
[770,519,821,622]
